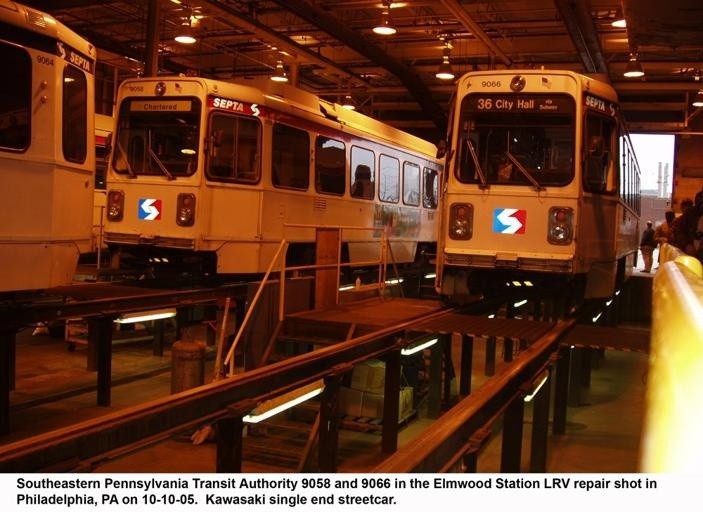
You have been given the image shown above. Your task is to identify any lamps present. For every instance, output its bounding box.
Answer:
[173,0,703,111]
[111,272,622,425]
[180,133,197,155]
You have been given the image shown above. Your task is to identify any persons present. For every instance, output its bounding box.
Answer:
[650,189,703,266]
[639,219,657,274]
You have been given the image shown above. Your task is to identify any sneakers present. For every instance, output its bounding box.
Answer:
[641,269,650,273]
